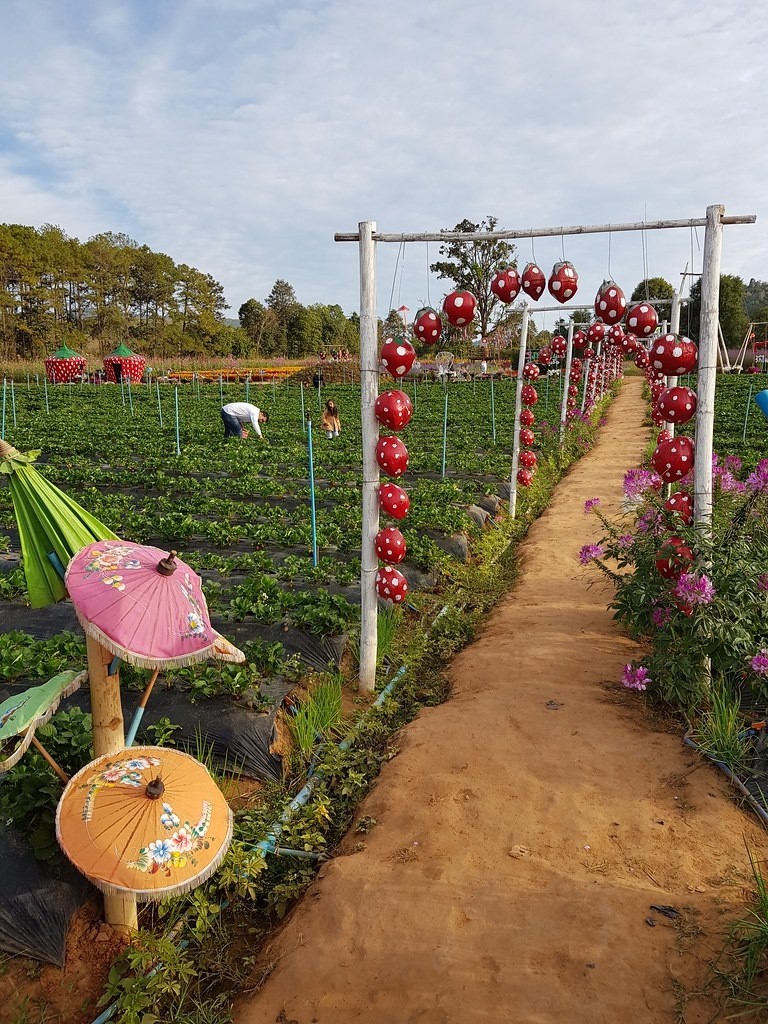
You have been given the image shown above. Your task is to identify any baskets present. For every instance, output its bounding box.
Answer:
[241,428,250,440]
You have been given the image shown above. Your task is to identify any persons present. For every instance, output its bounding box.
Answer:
[220,402,269,439]
[313,371,327,392]
[321,399,340,440]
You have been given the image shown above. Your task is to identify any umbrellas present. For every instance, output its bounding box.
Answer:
[0,440,123,609]
[0,669,89,786]
[55,745,234,901]
[64,540,216,670]
[125,627,245,748]
